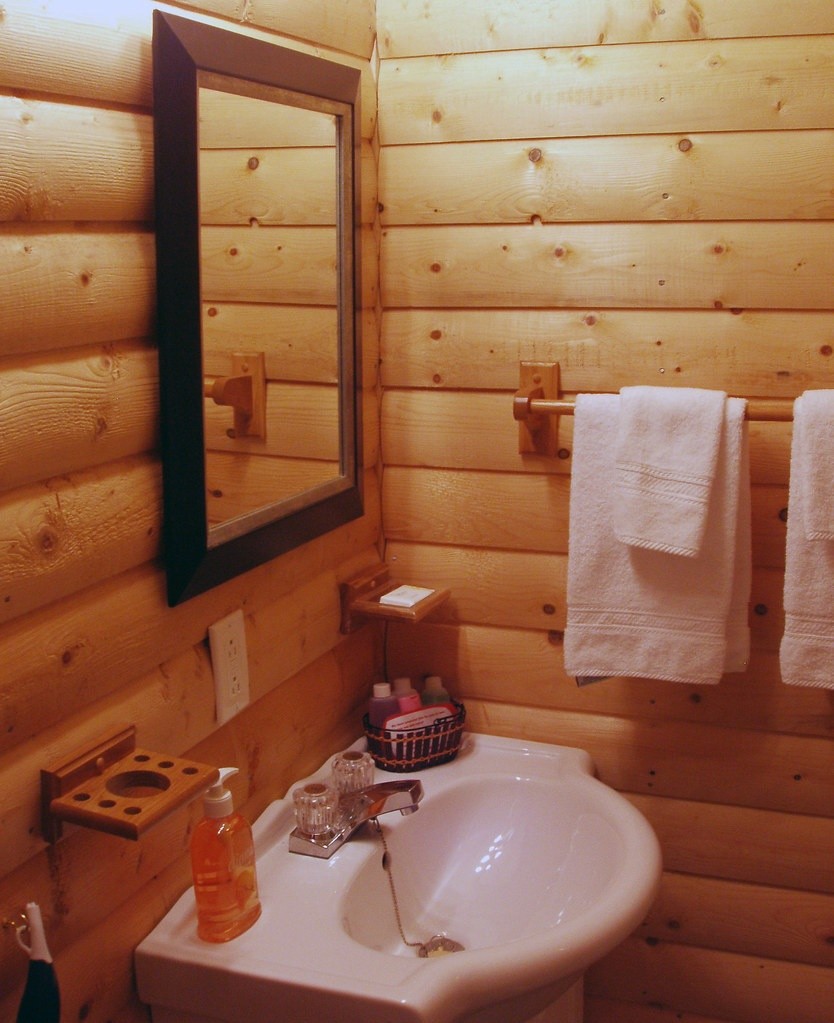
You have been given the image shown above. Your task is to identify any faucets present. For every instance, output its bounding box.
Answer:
[289,749,424,861]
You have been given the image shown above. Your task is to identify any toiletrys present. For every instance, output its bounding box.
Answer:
[188,768,263,944]
[369,674,452,755]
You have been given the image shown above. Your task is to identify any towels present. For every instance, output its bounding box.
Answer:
[562,385,751,688]
[780,384,834,693]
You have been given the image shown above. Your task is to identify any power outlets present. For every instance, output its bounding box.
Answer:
[208,611,253,725]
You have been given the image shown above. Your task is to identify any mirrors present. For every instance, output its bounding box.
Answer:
[153,6,366,609]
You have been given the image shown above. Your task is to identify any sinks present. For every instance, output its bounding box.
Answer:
[135,733,664,1022]
